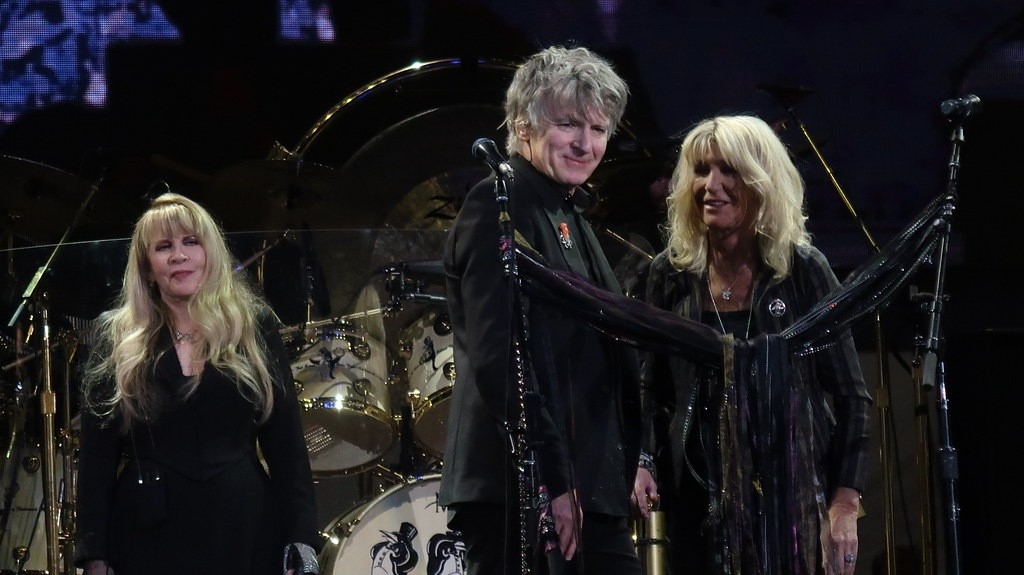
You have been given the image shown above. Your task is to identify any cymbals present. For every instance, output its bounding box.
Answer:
[0,152,144,249]
[206,159,380,245]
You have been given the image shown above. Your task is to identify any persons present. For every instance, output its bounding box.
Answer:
[435,45,646,575]
[629,111,874,575]
[71,191,321,575]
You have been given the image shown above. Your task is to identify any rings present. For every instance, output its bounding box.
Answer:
[844,554,856,567]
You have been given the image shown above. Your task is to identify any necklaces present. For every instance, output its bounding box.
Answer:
[172,326,202,345]
[713,260,749,301]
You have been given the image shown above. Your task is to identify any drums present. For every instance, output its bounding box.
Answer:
[622,498,674,575]
[1,434,83,574]
[324,470,474,575]
[391,296,456,459]
[286,309,405,473]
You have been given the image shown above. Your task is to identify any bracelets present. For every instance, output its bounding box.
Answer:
[638,452,658,481]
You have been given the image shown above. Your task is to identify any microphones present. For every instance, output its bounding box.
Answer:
[472,137,514,182]
[754,80,819,96]
[941,94,981,115]
